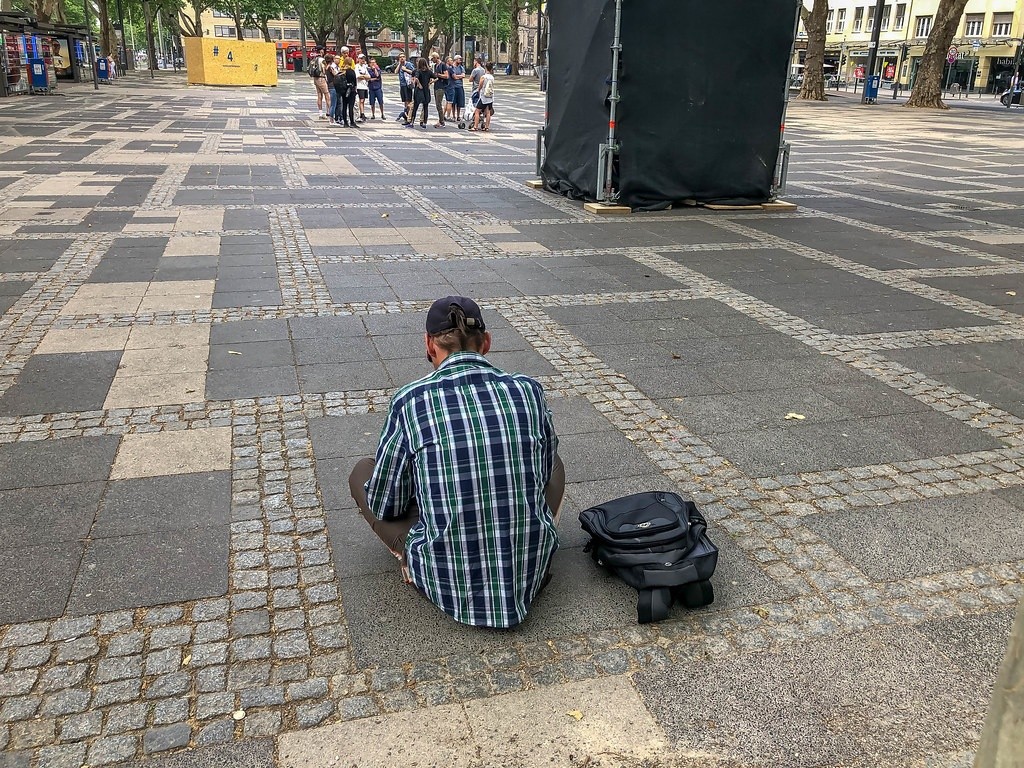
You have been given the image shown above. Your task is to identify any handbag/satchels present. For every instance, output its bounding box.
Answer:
[403,62,416,88]
[483,106,494,118]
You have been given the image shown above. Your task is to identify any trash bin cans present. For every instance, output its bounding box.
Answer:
[97,58,108,78]
[949,83,960,94]
[1019,87,1024,105]
[866,75,880,98]
[28,58,48,87]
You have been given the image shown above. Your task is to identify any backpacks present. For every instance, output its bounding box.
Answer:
[308,56,321,77]
[333,70,352,95]
[578,491,719,624]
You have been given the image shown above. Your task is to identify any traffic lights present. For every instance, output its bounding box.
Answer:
[900,42,910,62]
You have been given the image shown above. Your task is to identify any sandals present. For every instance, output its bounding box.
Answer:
[481,127,489,131]
[468,126,478,131]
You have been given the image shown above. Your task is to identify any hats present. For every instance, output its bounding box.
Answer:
[315,45,325,51]
[426,296,485,362]
[429,52,439,59]
[358,54,366,58]
[453,55,462,60]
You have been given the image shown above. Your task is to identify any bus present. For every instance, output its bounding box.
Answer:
[285,45,356,70]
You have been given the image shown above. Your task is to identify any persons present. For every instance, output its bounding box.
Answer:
[395,52,495,131]
[1010,72,1019,90]
[312,45,386,127]
[348,296,566,629]
[107,52,116,78]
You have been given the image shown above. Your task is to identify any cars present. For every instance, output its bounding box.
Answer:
[385,62,399,74]
[1000,88,1024,107]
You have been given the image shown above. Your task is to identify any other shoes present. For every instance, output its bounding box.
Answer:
[452,117,456,122]
[435,123,445,128]
[406,124,415,129]
[344,123,349,128]
[402,121,410,125]
[318,111,366,124]
[349,122,360,128]
[420,122,426,128]
[371,116,375,119]
[456,117,461,122]
[447,117,452,122]
[382,116,386,120]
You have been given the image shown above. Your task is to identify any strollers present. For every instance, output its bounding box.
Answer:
[456,88,487,131]
